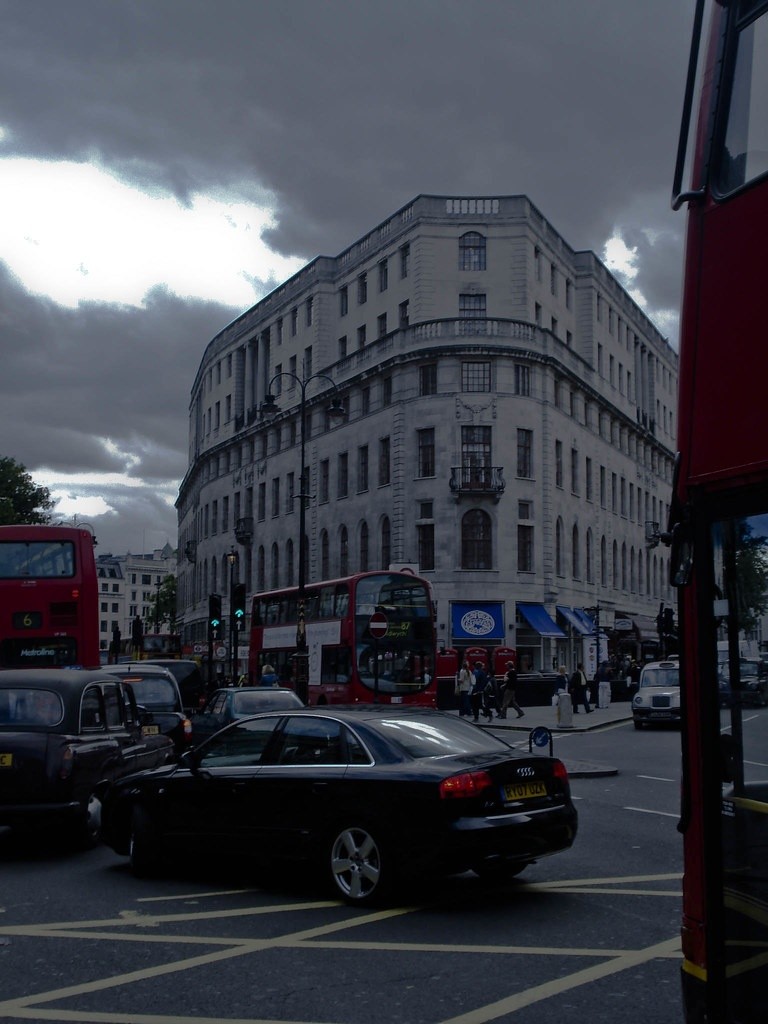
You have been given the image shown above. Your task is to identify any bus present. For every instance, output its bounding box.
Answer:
[108,633,181,671]
[657,0,768,1023]
[0,525,101,672]
[247,570,447,713]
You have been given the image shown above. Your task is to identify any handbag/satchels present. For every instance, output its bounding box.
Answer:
[454,686,460,695]
[552,693,559,708]
[586,689,591,702]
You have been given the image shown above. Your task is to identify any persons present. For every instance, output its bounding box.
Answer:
[255,664,280,687]
[553,659,642,714]
[457,661,525,723]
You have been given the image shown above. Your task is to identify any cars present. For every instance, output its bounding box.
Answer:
[100,701,578,908]
[0,664,177,852]
[187,686,306,745]
[714,653,768,710]
[626,660,682,731]
[78,661,193,757]
[119,659,207,718]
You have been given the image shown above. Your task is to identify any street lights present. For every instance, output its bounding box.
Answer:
[224,545,238,678]
[154,582,164,634]
[261,371,348,705]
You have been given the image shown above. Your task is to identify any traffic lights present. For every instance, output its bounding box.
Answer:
[230,582,246,632]
[207,592,222,641]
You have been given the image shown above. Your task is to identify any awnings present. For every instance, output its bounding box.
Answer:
[451,603,609,640]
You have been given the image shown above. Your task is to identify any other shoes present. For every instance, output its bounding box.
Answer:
[472,719,478,723]
[574,711,579,713]
[586,710,594,713]
[516,714,524,718]
[481,713,487,718]
[488,715,493,722]
[499,716,506,719]
[494,714,500,718]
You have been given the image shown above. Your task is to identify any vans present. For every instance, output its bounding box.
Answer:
[715,638,763,676]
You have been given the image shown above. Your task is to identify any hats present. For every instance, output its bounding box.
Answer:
[505,661,513,666]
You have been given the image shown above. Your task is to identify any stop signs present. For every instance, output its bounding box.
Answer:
[368,611,390,640]
